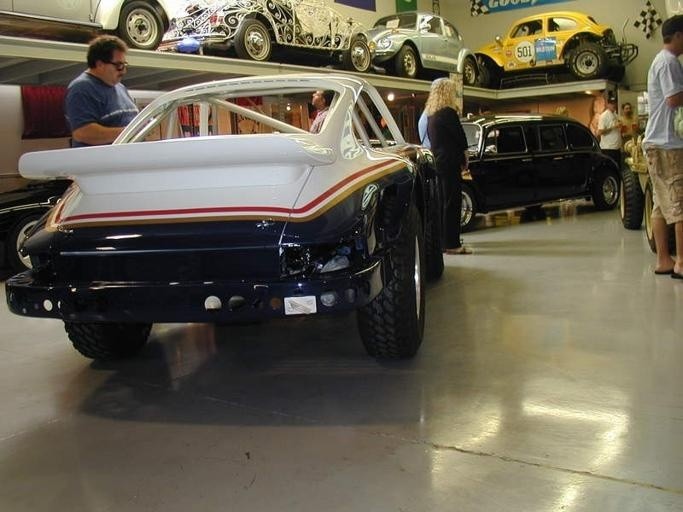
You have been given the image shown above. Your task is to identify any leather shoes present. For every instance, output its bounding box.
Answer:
[440,246,476,255]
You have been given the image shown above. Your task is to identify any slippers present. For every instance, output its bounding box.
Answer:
[654,268,673,275]
[671,274,682,279]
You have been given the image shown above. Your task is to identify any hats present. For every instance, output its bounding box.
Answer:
[607,96,616,103]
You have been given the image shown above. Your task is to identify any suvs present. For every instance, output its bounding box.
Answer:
[616,105,683,253]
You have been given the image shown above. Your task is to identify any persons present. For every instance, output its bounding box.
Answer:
[63,34,140,147]
[598,96,623,168]
[619,102,638,148]
[418,109,433,152]
[641,15,683,278]
[427,78,474,255]
[308,91,335,133]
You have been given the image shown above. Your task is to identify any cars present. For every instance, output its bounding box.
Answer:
[7,74,444,376]
[1,161,81,274]
[0,0,173,49]
[160,1,377,75]
[362,11,481,85]
[453,114,623,231]
[469,10,630,83]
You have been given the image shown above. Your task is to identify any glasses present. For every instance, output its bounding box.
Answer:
[102,59,128,71]
